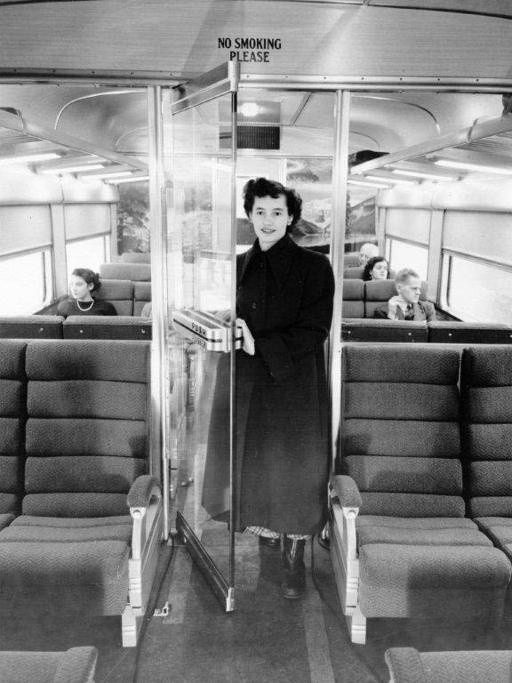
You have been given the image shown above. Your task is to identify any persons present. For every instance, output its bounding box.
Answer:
[198,176,333,600]
[358,243,382,270]
[359,256,391,280]
[370,268,435,322]
[57,265,118,314]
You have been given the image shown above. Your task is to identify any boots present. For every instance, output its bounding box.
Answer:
[279,535,307,601]
[254,535,288,605]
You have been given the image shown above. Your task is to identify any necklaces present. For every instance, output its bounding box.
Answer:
[77,298,97,312]
